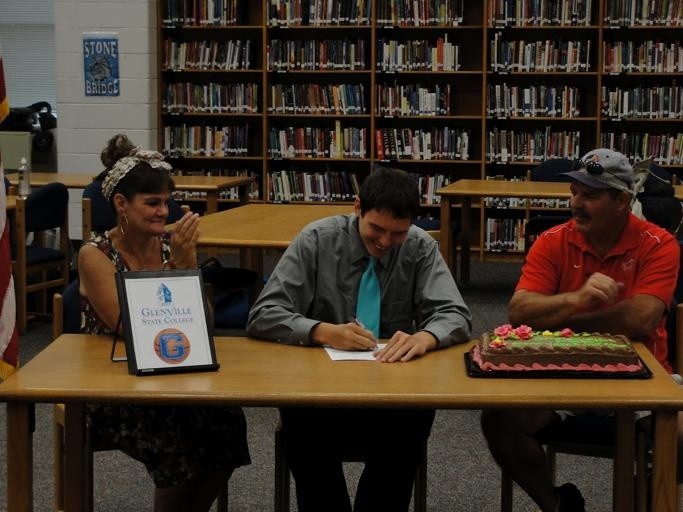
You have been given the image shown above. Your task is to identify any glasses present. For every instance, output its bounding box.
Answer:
[570,158,631,187]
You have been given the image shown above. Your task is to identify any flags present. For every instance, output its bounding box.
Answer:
[0,56,18,393]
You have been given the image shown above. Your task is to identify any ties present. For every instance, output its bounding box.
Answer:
[356,255,381,339]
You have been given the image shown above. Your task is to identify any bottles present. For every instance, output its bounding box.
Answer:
[17,155,32,200]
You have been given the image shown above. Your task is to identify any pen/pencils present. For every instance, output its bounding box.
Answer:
[351,314,379,349]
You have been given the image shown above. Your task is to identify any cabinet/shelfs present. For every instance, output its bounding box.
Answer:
[147,0,683,263]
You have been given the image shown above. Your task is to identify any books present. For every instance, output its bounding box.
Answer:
[600,86,679,185]
[602,2,680,73]
[373,80,472,205]
[486,1,592,74]
[374,1,466,73]
[266,84,368,203]
[158,0,252,70]
[266,2,369,72]
[484,174,572,253]
[485,82,580,162]
[162,80,261,200]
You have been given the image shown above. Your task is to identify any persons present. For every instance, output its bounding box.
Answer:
[76,133,252,512]
[243,167,473,512]
[81,168,116,234]
[480,144,681,509]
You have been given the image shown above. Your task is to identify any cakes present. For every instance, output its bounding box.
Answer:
[463,324,654,381]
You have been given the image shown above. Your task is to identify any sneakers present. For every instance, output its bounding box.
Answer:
[552,482,584,511]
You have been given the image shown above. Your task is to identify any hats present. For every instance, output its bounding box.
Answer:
[556,148,634,194]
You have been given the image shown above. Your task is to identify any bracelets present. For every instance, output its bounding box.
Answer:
[160,260,168,270]
